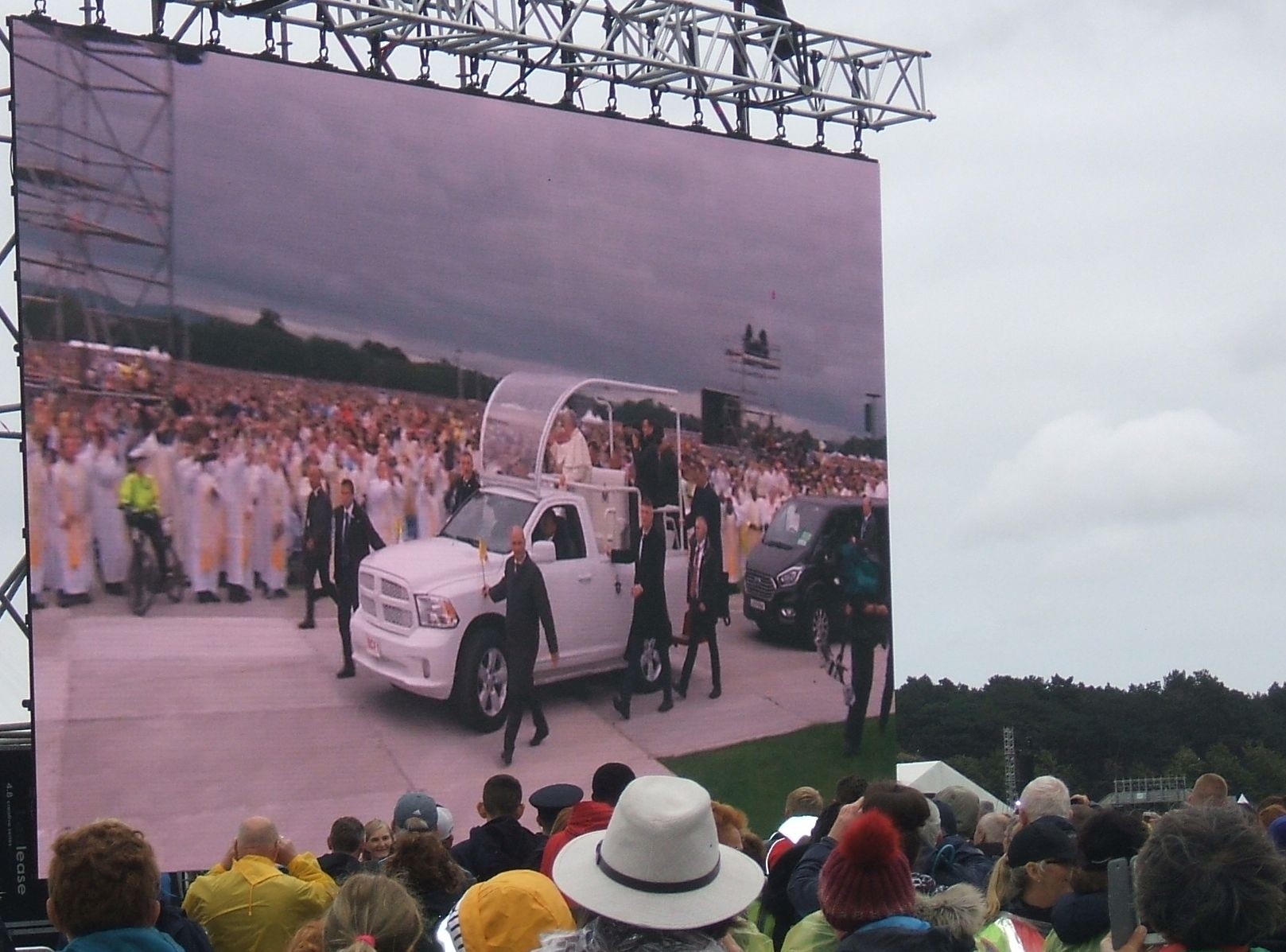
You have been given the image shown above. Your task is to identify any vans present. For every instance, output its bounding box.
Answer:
[745,496,891,653]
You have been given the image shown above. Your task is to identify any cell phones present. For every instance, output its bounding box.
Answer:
[1129,856,1167,944]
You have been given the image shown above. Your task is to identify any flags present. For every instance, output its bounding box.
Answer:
[479,537,488,561]
[394,518,403,537]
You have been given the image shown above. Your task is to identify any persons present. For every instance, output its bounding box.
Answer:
[25,340,894,763]
[48,760,1286,952]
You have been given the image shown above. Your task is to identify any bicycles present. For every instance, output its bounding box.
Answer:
[119,506,190,620]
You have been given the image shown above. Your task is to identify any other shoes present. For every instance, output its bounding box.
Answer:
[31,569,331,609]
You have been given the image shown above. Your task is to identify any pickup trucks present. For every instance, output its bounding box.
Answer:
[346,372,700,734]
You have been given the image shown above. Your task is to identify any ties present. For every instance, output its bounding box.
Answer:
[514,563,520,573]
[641,534,647,560]
[690,546,701,600]
[308,491,314,520]
[343,512,349,538]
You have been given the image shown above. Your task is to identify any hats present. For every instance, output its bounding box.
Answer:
[552,774,764,930]
[436,806,454,840]
[934,784,980,833]
[1007,815,1078,867]
[394,793,438,832]
[816,810,916,933]
[528,783,584,819]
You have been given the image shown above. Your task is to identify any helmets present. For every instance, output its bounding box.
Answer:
[126,448,148,466]
